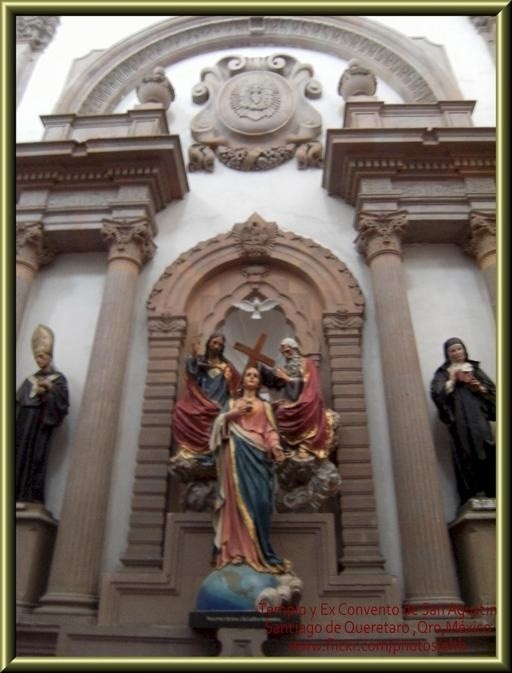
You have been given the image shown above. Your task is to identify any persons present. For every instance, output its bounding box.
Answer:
[172,333,332,573]
[16,352,69,505]
[431,337,496,506]
